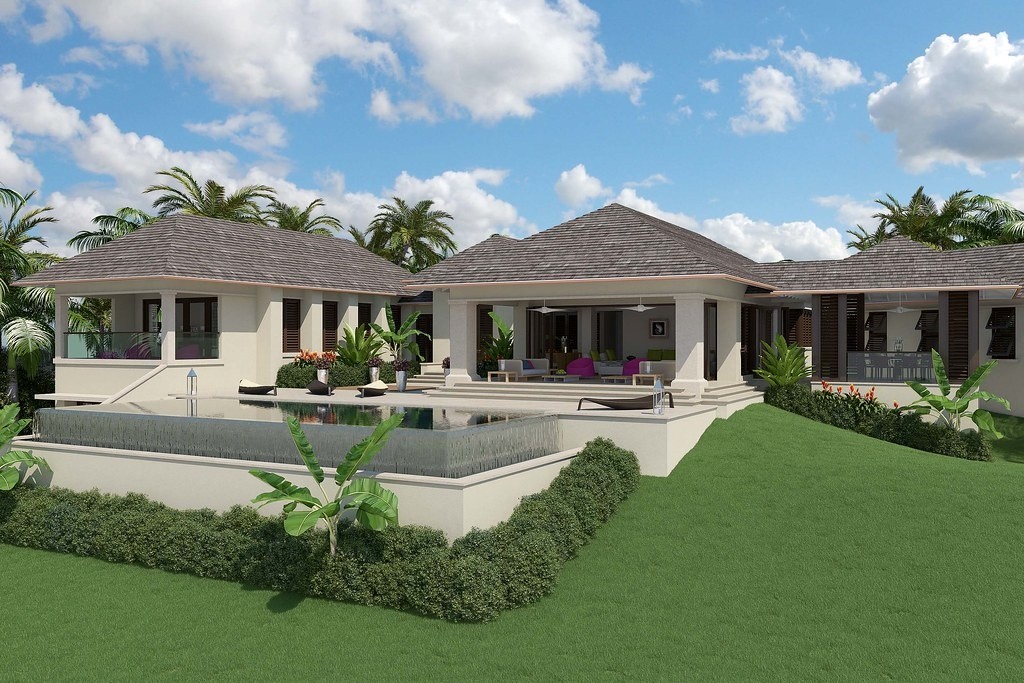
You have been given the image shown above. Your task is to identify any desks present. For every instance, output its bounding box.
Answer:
[598,366,623,380]
[632,374,664,387]
[488,372,518,383]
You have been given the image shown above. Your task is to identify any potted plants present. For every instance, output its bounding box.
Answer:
[392,358,409,391]
[442,357,450,375]
[368,357,384,382]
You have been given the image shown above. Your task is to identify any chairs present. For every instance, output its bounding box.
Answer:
[577,391,675,410]
[125,344,152,359]
[177,344,202,358]
[566,358,596,378]
[306,380,335,394]
[356,379,388,395]
[624,357,648,376]
[864,354,933,381]
[239,379,279,396]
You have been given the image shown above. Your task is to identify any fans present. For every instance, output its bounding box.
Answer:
[618,297,656,313]
[527,300,567,313]
[882,292,918,313]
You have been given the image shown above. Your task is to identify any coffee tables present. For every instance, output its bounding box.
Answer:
[542,375,581,383]
[601,376,632,384]
[519,374,544,382]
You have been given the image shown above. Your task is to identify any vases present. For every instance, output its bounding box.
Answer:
[894,337,904,352]
[317,368,329,384]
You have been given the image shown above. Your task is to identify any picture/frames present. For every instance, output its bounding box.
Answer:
[648,319,670,339]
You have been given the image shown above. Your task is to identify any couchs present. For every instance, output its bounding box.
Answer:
[498,359,550,381]
[638,360,676,385]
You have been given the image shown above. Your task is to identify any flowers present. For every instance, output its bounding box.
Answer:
[294,349,337,370]
[556,369,566,375]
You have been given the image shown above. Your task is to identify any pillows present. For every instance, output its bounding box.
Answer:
[662,349,675,360]
[522,360,534,369]
[647,348,662,361]
[590,350,600,361]
[599,353,608,361]
[605,348,617,360]
